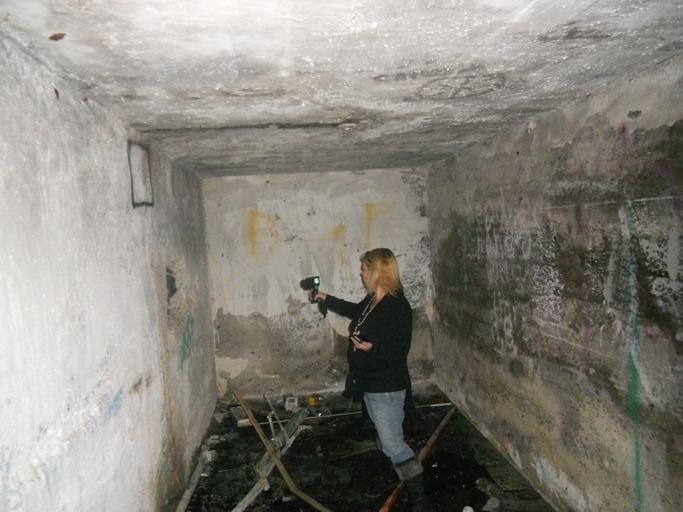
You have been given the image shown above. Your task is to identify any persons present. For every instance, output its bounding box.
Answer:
[308,248,426,505]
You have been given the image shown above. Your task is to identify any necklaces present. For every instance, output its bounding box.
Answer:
[352,296,376,352]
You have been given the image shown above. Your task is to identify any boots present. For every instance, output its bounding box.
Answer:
[364,457,400,498]
[403,474,429,512]
